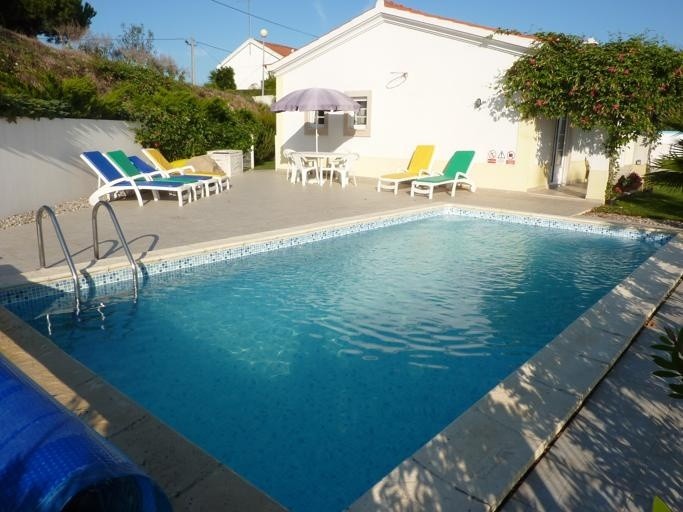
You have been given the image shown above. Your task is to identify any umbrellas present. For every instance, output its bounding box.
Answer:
[269,87,361,179]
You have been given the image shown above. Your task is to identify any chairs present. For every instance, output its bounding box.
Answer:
[376,144,477,200]
[80,148,230,207]
[281,149,359,188]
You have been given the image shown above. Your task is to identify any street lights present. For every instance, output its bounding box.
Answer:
[257,25,269,102]
